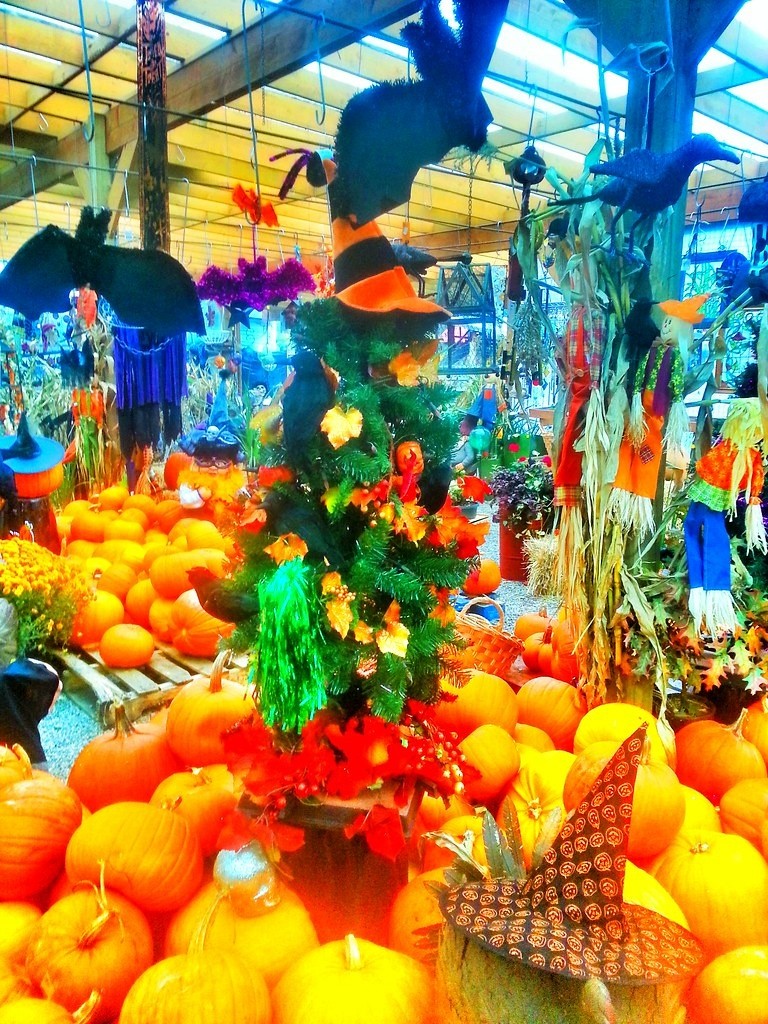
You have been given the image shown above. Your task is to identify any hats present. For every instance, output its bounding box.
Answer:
[0,410,64,474]
[179,354,247,468]
[657,294,714,323]
[437,722,708,985]
[320,156,454,325]
[463,386,484,419]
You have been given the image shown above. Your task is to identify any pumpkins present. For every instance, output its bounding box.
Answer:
[55,452,581,682]
[0,649,768,1024]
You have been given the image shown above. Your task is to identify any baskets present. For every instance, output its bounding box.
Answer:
[440,596,525,678]
